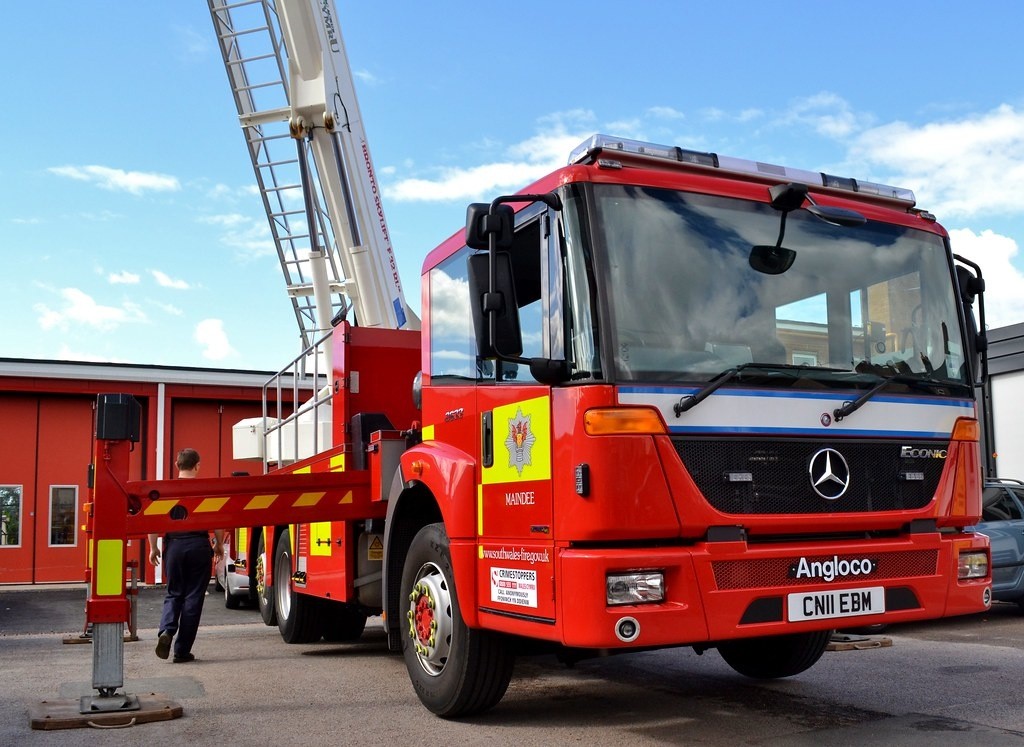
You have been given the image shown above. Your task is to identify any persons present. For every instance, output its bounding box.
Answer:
[149,448,224,662]
[57,528,67,544]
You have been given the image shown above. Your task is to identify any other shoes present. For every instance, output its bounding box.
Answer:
[155,629,172,659]
[173,653,194,663]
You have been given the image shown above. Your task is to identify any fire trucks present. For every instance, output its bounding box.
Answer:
[23,0,991,736]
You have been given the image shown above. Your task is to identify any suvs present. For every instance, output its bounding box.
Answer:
[962,475,1024,614]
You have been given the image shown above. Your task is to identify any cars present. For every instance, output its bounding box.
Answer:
[210,532,249,610]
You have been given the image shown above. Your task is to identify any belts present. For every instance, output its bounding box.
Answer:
[167,537,206,541]
